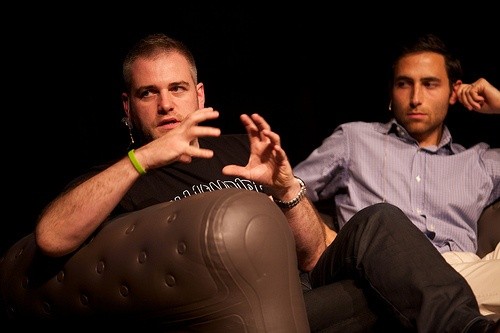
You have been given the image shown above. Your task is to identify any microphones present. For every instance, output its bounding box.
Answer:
[122,116,136,146]
[389,98,394,113]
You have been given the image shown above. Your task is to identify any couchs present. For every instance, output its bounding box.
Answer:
[0,188,500,333]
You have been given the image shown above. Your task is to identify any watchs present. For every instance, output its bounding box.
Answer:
[271,174,307,208]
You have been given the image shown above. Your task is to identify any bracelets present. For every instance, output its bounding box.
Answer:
[127,149,146,176]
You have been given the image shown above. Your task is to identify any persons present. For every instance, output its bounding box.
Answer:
[36,32,500,332]
[290,43,499,323]
